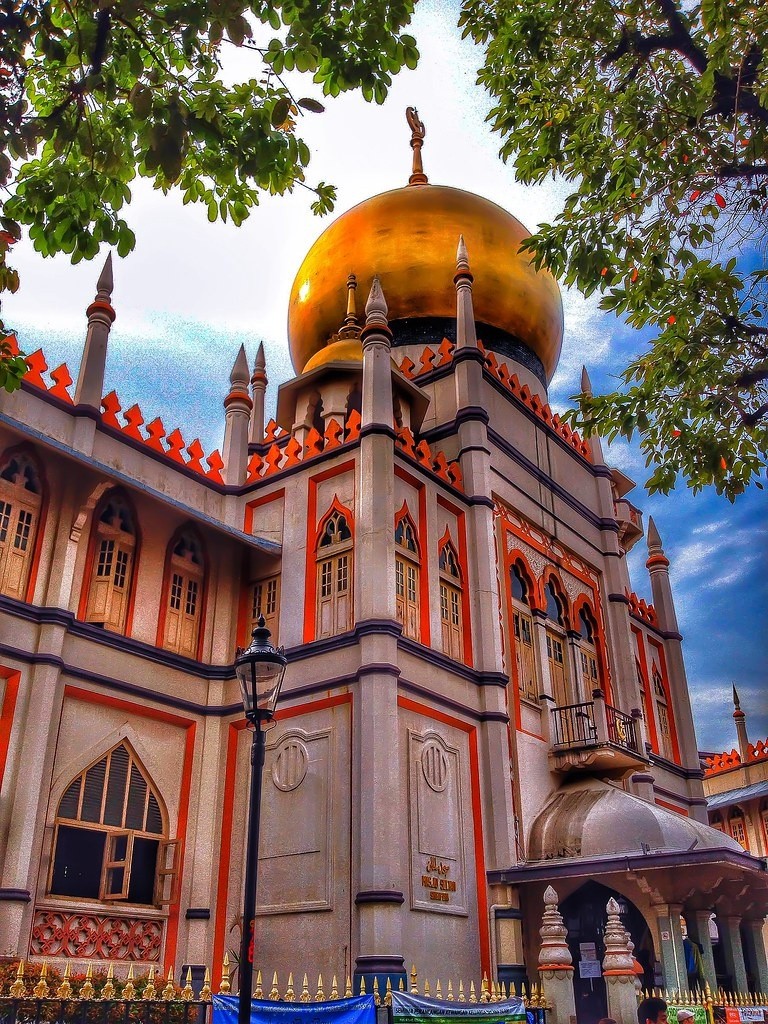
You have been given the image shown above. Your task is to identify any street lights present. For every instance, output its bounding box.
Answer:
[233,614,288,1024]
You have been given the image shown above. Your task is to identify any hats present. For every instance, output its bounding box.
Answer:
[677,1009,695,1021]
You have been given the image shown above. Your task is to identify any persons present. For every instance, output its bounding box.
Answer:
[598,1018,619,1024]
[677,1010,696,1024]
[638,997,669,1024]
